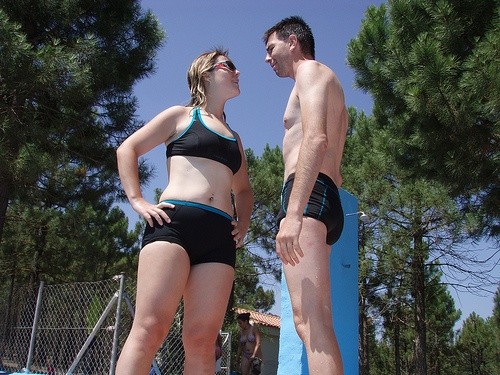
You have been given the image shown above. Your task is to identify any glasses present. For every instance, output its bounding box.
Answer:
[210,60,236,72]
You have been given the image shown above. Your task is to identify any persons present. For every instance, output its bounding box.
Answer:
[215,333,223,372]
[235,313,263,375]
[262,16,348,375]
[115,51,254,375]
